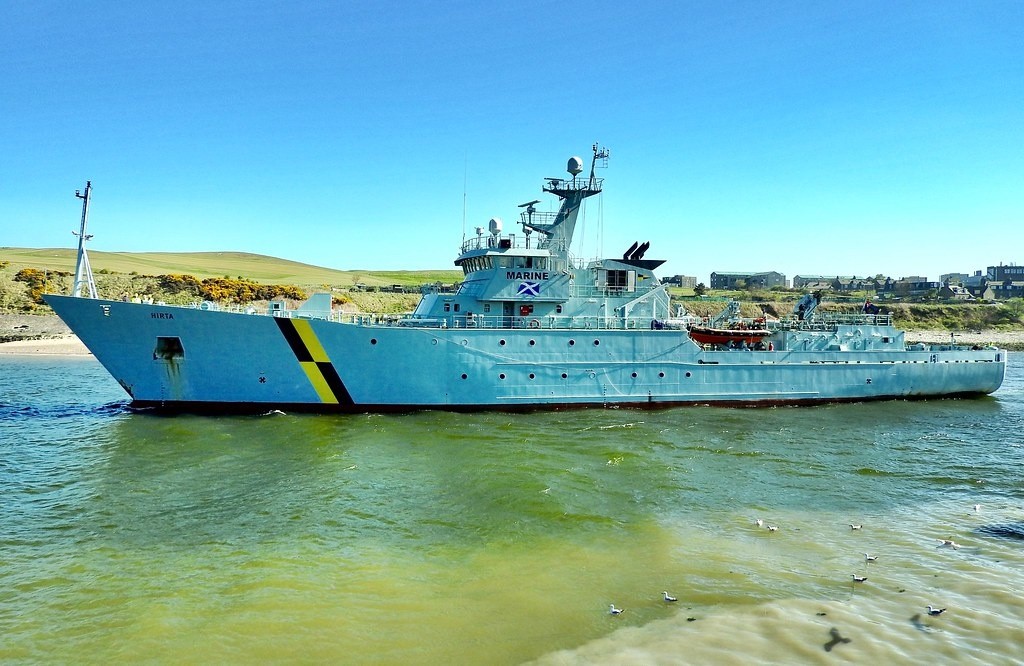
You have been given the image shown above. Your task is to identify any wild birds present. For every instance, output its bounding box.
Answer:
[925,605,946,616]
[661,590,678,602]
[756,518,779,531]
[864,553,878,561]
[608,603,625,616]
[850,574,867,582]
[848,524,862,530]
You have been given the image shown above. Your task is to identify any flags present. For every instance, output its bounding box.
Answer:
[863,299,879,314]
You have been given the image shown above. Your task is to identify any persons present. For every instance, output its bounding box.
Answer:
[686,323,691,331]
[713,338,775,351]
[122,292,156,305]
[727,322,762,330]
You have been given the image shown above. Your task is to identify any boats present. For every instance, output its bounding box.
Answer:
[689,313,772,344]
[39,140,1007,416]
